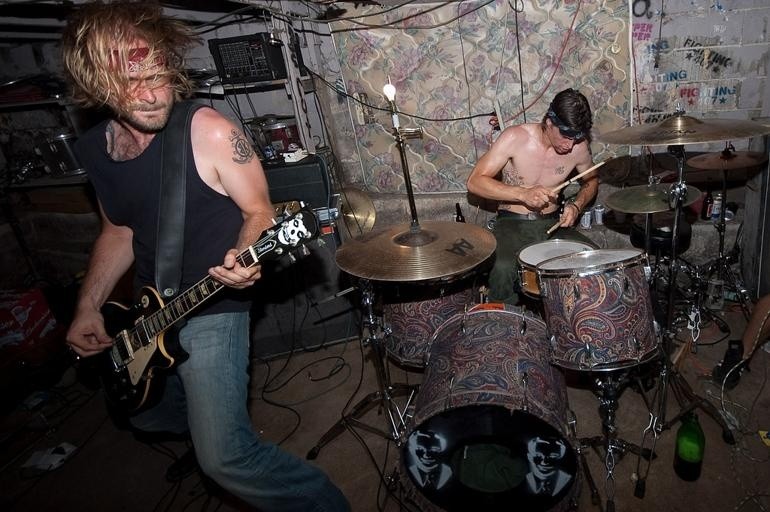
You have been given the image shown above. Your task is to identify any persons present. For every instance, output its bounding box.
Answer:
[467,88,605,312]
[61,2,358,512]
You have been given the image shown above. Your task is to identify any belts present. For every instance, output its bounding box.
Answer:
[497,209,561,221]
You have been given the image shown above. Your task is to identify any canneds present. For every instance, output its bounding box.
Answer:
[710,194,723,221]
[487,216,497,229]
[580,211,591,229]
[453,214,462,222]
[287,143,299,151]
[265,146,278,160]
[595,205,605,225]
[725,202,738,221]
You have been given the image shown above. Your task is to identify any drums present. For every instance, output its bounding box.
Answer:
[516,238,595,302]
[394,305,581,512]
[627,209,691,255]
[379,266,488,373]
[534,247,661,374]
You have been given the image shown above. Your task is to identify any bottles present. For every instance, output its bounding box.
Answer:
[702,187,713,220]
[455,203,466,224]
[671,413,706,482]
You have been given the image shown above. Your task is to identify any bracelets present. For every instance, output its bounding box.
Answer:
[569,201,581,213]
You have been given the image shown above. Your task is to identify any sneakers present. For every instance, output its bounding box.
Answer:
[712,340,749,390]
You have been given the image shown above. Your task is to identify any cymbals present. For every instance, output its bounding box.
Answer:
[605,183,701,214]
[687,148,769,170]
[337,188,377,242]
[335,219,499,284]
[597,115,770,145]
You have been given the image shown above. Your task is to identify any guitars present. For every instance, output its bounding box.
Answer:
[96,198,327,419]
